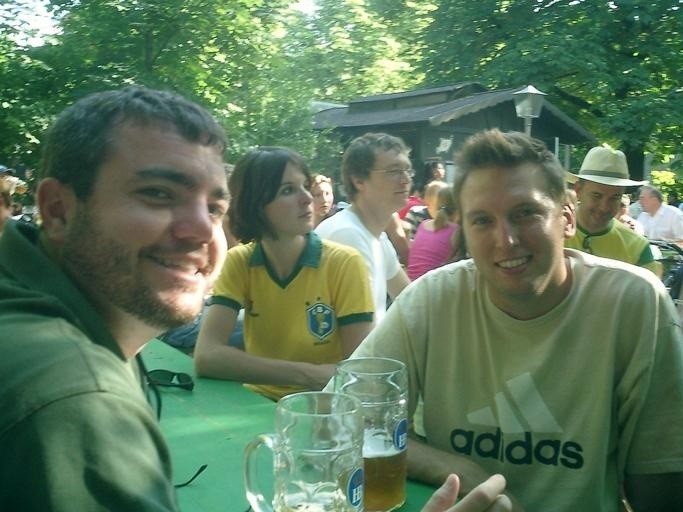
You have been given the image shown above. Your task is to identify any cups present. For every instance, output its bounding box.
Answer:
[244,392,365,512]
[332,358,408,512]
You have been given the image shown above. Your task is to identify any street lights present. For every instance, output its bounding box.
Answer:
[510,84,548,136]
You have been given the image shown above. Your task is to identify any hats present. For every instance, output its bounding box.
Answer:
[564,146,646,190]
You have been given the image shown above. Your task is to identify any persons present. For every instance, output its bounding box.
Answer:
[385,212,410,264]
[0,192,13,237]
[317,127,683,512]
[10,203,25,221]
[613,194,644,235]
[309,173,339,232]
[420,161,445,199]
[194,147,375,403]
[637,187,683,248]
[407,186,462,282]
[564,145,664,280]
[402,180,447,239]
[398,185,426,219]
[156,162,246,349]
[0,86,512,512]
[0,165,19,196]
[314,132,411,327]
[668,193,683,212]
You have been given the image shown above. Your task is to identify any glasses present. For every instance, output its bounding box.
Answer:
[172,463,252,510]
[362,166,417,180]
[145,369,194,392]
